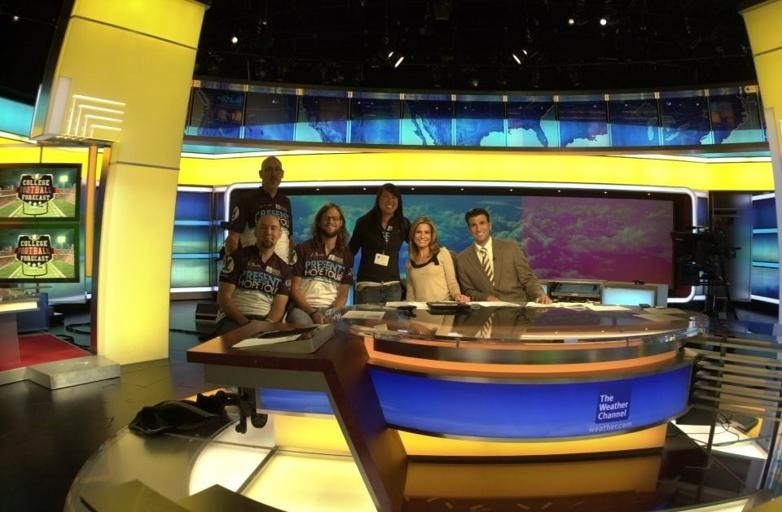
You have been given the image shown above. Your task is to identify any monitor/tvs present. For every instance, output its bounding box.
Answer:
[0,223,80,283]
[0,163,83,222]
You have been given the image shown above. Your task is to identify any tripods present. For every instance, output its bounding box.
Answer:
[693,249,739,325]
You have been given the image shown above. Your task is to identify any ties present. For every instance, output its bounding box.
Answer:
[480,248,494,285]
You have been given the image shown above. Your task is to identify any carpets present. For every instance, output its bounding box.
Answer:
[0,334,91,373]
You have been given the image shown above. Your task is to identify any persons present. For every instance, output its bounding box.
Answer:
[404,219,470,306]
[223,154,298,267]
[347,183,457,317]
[216,212,295,331]
[360,303,549,346]
[454,207,555,304]
[286,201,359,326]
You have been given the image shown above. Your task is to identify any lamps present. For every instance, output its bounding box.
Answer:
[371,30,406,69]
[566,1,623,27]
[510,28,545,66]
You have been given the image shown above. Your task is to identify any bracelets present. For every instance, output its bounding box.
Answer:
[309,310,318,319]
[264,318,274,323]
